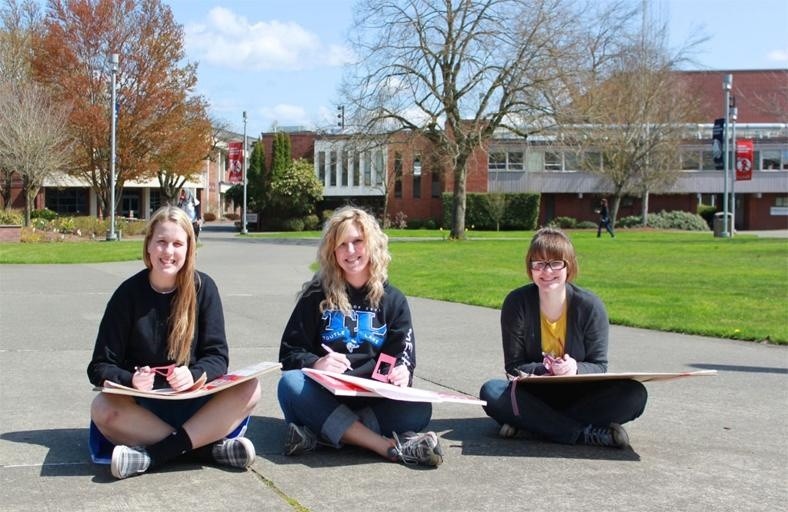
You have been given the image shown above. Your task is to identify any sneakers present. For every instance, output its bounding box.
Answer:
[586,423,629,450]
[111,445,152,479]
[284,421,318,456]
[388,430,443,467]
[213,437,254,469]
[500,423,539,441]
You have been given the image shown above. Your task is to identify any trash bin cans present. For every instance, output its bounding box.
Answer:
[713,212,732,237]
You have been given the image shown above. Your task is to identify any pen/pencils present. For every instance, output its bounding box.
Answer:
[166,364,174,376]
[320,343,355,372]
[133,366,143,374]
[542,352,558,365]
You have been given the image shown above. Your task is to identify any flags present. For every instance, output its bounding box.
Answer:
[712,117,727,172]
[227,142,244,185]
[734,138,754,182]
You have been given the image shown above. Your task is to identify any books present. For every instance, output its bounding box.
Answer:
[505,372,718,385]
[90,356,283,401]
[302,365,489,407]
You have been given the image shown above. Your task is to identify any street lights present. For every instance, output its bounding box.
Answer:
[106,54,120,241]
[241,112,248,235]
[720,74,737,240]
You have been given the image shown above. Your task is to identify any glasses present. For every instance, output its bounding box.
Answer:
[530,259,567,269]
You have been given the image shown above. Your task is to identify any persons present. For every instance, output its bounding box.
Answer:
[275,204,444,470]
[82,204,264,479]
[178,189,202,244]
[593,198,616,238]
[478,226,648,451]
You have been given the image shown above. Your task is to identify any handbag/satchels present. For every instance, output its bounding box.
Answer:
[600,218,610,225]
